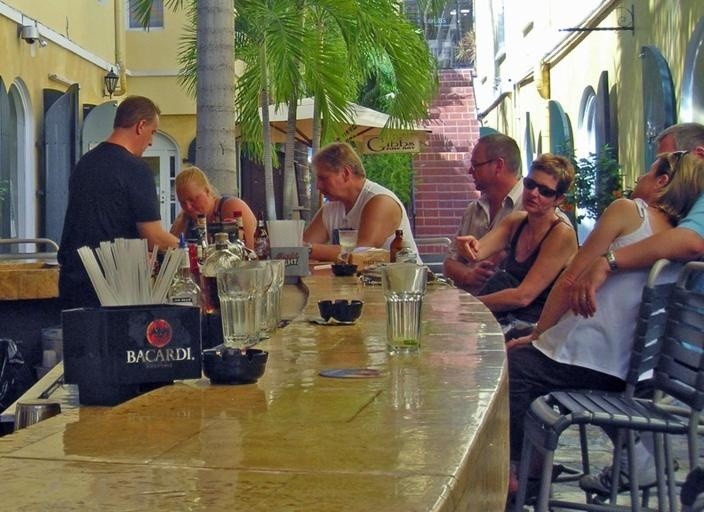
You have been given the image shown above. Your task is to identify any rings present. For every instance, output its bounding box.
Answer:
[579,293,586,300]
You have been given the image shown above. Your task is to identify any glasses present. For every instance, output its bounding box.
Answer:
[522,177,562,198]
[669,150,690,182]
[471,155,504,172]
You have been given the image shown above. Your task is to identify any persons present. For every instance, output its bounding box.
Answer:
[504,147,704,500]
[452,154,580,346]
[565,123,704,496]
[166,164,258,253]
[443,134,575,300]
[261,142,423,268]
[56,94,180,309]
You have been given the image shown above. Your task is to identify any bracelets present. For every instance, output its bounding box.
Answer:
[306,241,312,260]
[531,321,543,335]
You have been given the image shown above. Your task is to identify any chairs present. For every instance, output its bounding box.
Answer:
[603,255,681,512]
[513,256,702,512]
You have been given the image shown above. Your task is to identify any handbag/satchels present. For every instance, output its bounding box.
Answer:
[475,269,519,318]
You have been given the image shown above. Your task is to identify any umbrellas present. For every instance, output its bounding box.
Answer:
[231,90,431,218]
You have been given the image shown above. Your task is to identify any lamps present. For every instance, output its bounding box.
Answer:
[535,4,637,40]
[18,25,48,51]
[97,64,117,100]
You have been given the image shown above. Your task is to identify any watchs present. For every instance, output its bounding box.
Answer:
[600,250,618,273]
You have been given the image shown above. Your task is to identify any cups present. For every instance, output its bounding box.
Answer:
[14,399,59,432]
[379,263,427,349]
[217,257,286,349]
[338,230,359,259]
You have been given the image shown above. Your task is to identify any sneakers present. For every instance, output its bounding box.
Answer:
[579,449,661,496]
[642,430,682,472]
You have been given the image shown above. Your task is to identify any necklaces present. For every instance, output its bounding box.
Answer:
[524,214,555,252]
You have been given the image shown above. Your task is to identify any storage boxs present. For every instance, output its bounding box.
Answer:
[268,242,313,276]
[57,296,206,389]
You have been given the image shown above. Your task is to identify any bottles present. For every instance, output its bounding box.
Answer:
[151,231,257,315]
[253,210,269,258]
[195,211,206,243]
[389,227,408,263]
[232,209,245,245]
